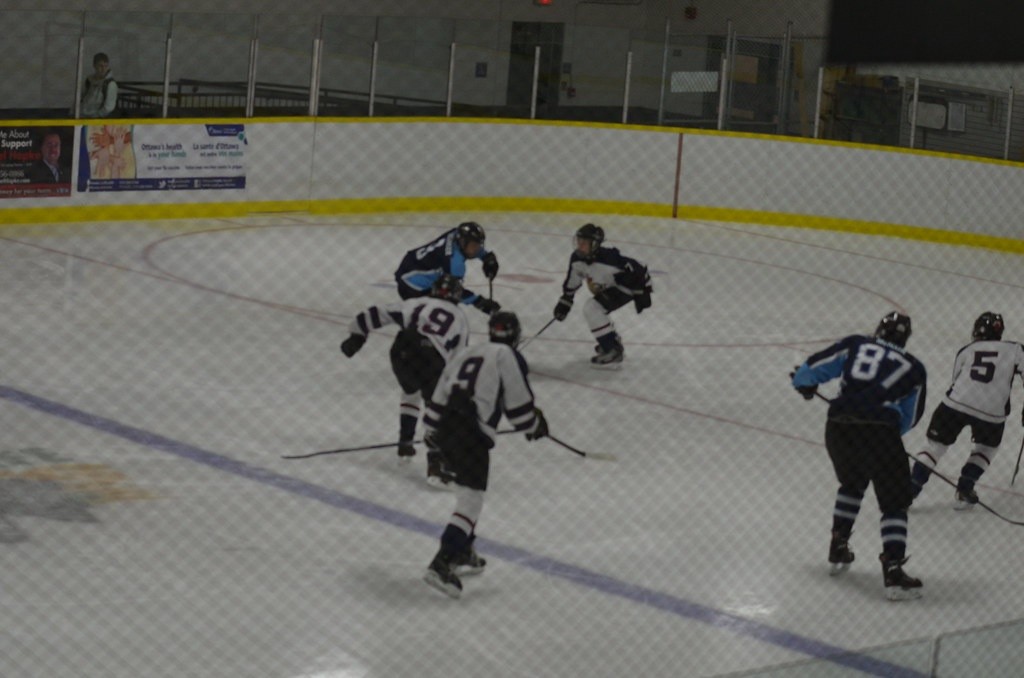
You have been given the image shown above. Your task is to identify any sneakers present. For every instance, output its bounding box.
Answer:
[395,440,418,466]
[451,546,486,578]
[426,462,458,491]
[422,558,464,599]
[593,337,621,355]
[828,531,856,573]
[878,556,924,600]
[954,476,979,513]
[589,348,623,370]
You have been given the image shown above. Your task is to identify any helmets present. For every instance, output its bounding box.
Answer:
[874,309,911,347]
[431,274,464,306]
[489,311,520,345]
[456,221,485,259]
[575,224,606,245]
[971,312,1004,342]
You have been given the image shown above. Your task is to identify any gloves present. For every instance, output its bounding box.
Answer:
[797,383,821,400]
[472,295,501,315]
[527,417,551,443]
[483,253,498,280]
[553,304,570,322]
[337,332,367,358]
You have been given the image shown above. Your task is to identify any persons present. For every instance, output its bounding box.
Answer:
[30,132,66,183]
[395,221,502,313]
[911,312,1024,510]
[81,52,119,117]
[422,311,549,599]
[342,273,470,490]
[793,311,928,600]
[88,126,132,178]
[554,223,652,371]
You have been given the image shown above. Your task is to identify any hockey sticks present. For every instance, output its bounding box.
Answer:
[489,276,492,299]
[546,432,623,461]
[814,390,1024,526]
[1011,438,1024,484]
[517,314,561,353]
[280,429,520,461]
